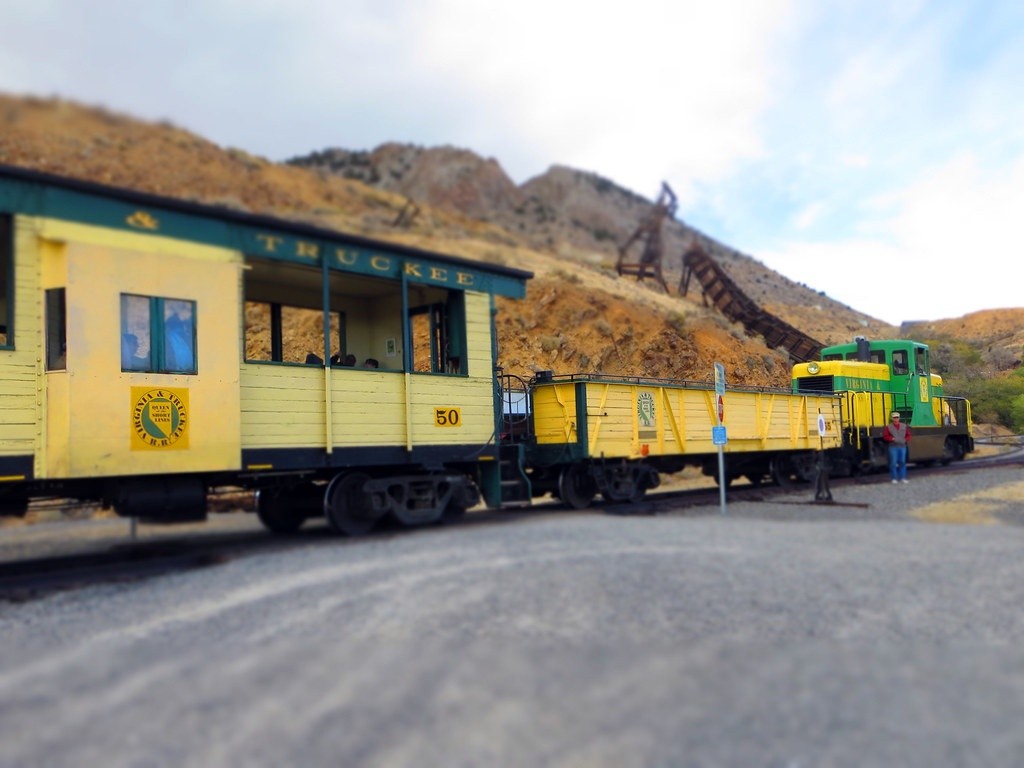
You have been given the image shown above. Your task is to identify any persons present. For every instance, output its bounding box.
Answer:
[330,352,379,368]
[882,412,911,484]
[121,333,150,370]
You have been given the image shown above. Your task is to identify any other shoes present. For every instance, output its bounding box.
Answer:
[902,478,909,483]
[892,479,898,483]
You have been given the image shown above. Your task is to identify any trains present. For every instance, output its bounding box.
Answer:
[1,163,977,539]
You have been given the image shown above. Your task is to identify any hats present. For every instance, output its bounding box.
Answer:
[891,412,900,417]
